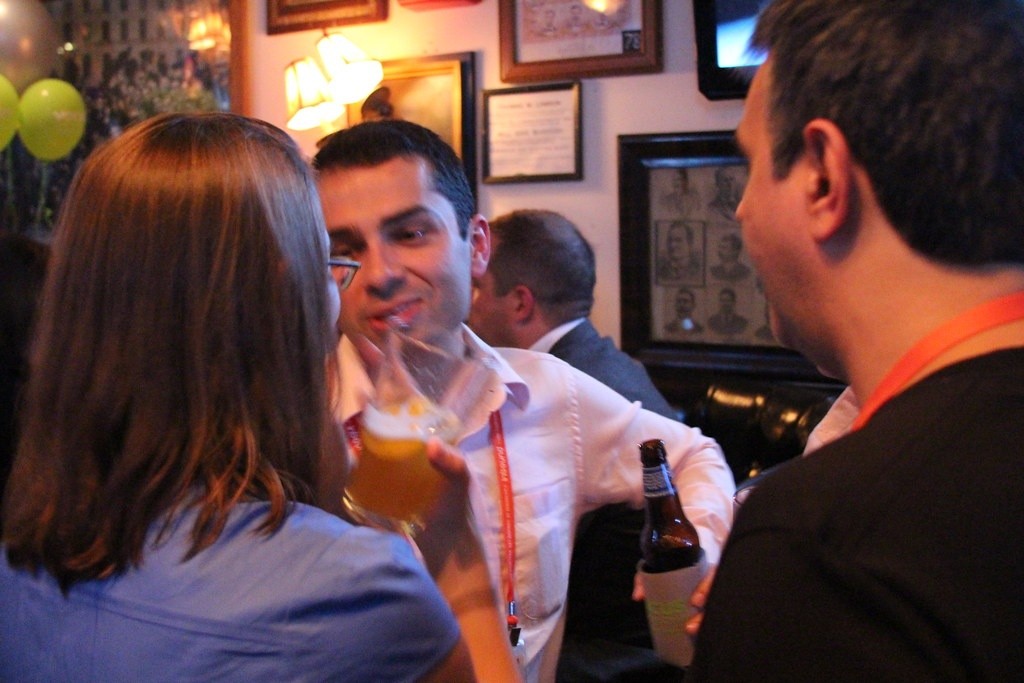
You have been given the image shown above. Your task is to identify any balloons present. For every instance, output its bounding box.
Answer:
[0,0,87,175]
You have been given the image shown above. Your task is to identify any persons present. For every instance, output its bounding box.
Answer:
[663,167,700,213]
[688,0,1024,683]
[665,290,703,336]
[659,224,701,282]
[707,165,742,220]
[303,120,737,683]
[712,234,750,283]
[0,112,521,683]
[709,290,748,334]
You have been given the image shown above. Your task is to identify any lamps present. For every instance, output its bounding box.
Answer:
[281,27,385,129]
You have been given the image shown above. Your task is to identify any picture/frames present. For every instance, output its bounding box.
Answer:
[617,131,820,377]
[482,83,583,185]
[341,51,479,213]
[498,0,664,83]
[264,1,388,36]
[41,1,251,124]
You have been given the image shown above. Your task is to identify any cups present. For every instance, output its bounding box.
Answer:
[342,315,481,539]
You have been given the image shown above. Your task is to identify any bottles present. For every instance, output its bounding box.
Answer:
[638,438,708,669]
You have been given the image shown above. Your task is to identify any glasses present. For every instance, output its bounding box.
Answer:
[325,252,360,290]
